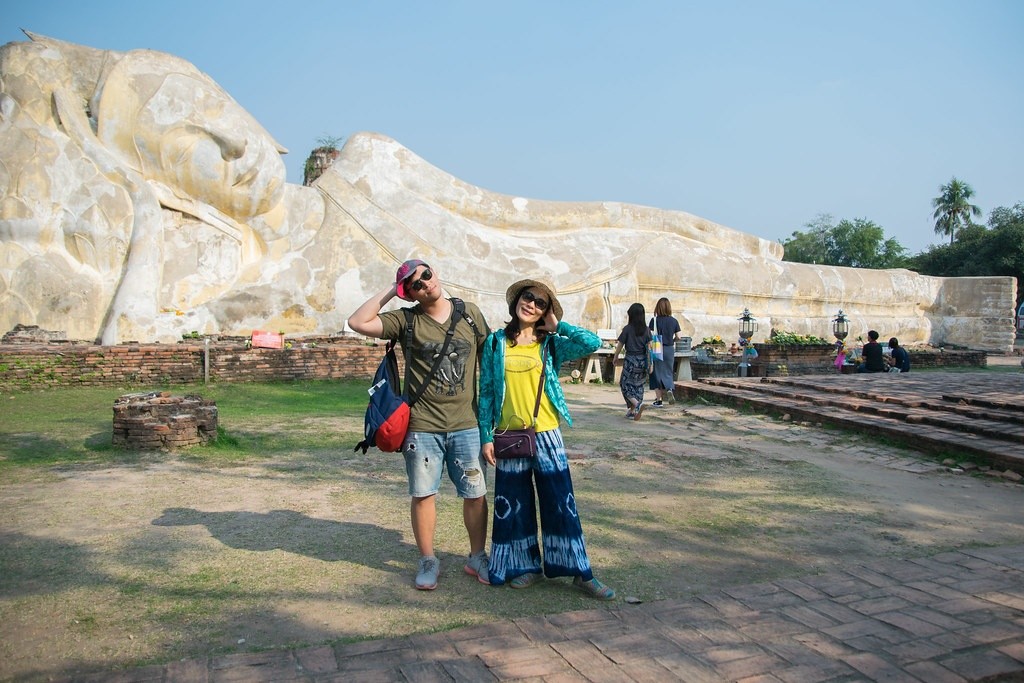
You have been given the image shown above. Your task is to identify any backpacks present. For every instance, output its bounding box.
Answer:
[364,299,414,452]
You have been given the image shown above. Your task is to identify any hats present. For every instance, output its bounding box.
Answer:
[396,259,430,302]
[506,278,563,321]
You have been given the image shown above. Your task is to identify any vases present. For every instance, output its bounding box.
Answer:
[745,347,758,358]
[703,344,722,353]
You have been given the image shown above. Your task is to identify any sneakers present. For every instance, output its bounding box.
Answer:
[634,402,645,421]
[666,388,675,405]
[464,550,491,585]
[625,410,635,420]
[415,557,440,590]
[511,572,546,589]
[653,401,663,407]
[572,575,616,600]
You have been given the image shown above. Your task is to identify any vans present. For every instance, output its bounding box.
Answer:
[1015,302,1024,333]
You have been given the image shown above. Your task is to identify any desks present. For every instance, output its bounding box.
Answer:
[609,351,698,383]
[578,349,617,384]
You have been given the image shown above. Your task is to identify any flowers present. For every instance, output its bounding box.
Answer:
[702,335,725,347]
[738,338,754,349]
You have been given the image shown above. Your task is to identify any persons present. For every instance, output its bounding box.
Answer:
[858,330,884,374]
[883,337,910,373]
[477,278,618,600]
[347,260,494,591]
[649,298,682,407]
[613,303,654,421]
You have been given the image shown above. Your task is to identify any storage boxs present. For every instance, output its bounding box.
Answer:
[596,328,617,339]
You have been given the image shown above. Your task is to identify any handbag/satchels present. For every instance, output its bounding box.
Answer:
[646,317,664,362]
[494,429,536,458]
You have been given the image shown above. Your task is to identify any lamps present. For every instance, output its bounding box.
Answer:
[832,309,850,338]
[737,308,758,338]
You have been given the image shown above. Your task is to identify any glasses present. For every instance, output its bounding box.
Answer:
[521,291,547,310]
[407,268,432,291]
